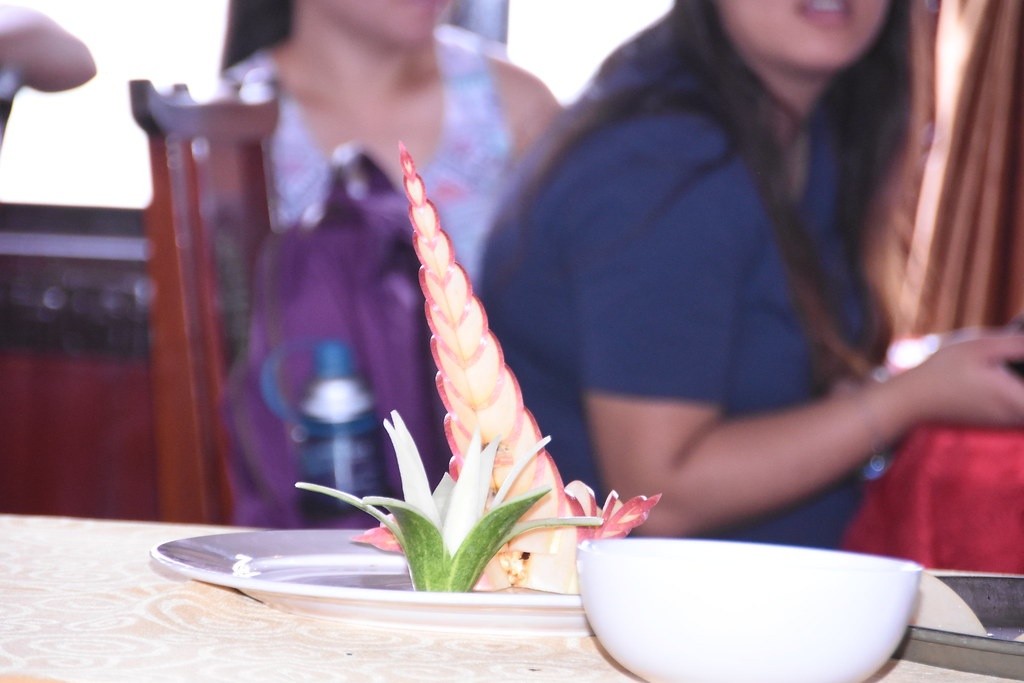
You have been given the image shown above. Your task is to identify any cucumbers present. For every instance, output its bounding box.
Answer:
[293,408,603,591]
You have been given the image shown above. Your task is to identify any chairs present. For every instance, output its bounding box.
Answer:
[129,77,280,524]
[224,154,446,524]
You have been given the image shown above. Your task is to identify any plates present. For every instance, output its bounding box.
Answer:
[155,526,585,627]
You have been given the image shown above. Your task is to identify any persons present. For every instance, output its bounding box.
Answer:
[211,0,564,281]
[475,0,1023,552]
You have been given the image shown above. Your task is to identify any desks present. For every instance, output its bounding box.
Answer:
[0,513,1024,683]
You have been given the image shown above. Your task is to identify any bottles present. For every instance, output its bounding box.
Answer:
[298,340,382,525]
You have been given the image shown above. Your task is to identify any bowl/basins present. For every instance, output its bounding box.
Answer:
[576,538,922,682]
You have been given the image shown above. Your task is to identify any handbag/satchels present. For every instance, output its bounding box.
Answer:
[841,422,1024,575]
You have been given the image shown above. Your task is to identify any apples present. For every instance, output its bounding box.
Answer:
[348,142,662,554]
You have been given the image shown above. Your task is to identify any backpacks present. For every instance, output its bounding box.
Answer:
[221,149,454,529]
